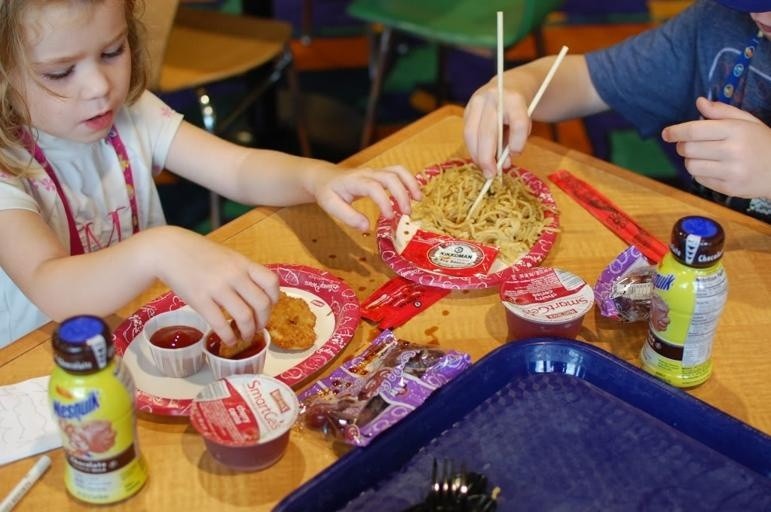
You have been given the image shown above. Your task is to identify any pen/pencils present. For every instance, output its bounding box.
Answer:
[0,454,52,512]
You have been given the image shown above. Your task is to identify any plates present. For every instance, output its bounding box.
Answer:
[111,262,361,420]
[373,157,561,292]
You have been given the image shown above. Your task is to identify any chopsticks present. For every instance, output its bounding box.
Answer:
[456,10,570,222]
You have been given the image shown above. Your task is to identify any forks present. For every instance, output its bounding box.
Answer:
[409,456,505,512]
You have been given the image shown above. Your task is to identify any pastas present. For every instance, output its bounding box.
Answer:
[406,164,562,271]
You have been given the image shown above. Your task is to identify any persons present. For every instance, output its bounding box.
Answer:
[1,0,423,351]
[462,1,771,229]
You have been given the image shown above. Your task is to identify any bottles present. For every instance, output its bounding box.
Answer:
[47,314,147,506]
[634,215,728,390]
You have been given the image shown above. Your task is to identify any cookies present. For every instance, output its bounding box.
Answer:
[218,318,261,358]
[267,290,319,354]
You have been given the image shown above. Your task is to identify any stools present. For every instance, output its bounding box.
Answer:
[132,0,313,231]
[345,0,560,151]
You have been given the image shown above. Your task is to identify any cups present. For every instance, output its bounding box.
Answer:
[498,267,599,341]
[201,324,269,379]
[140,312,207,379]
[188,373,297,470]
